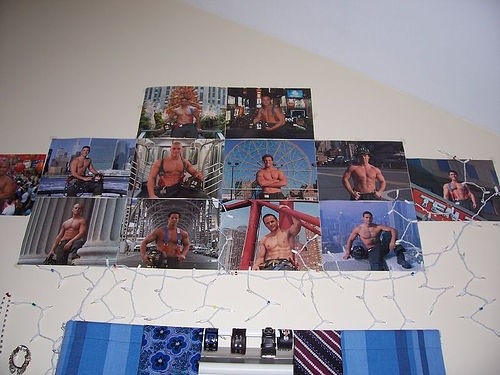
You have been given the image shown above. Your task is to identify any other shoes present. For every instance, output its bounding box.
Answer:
[397,259,412,269]
[72,252,81,260]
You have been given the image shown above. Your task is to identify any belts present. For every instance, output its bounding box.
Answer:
[367,247,375,252]
[267,260,294,269]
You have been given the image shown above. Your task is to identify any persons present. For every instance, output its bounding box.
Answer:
[147,141,209,199]
[141,211,190,269]
[344,211,412,271]
[48,202,88,263]
[343,146,385,201]
[256,155,286,199]
[67,146,103,195]
[0,158,43,216]
[251,205,302,271]
[442,170,477,213]
[168,97,201,137]
[252,94,286,131]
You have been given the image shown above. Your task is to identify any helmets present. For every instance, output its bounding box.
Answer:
[352,146,370,156]
[145,250,163,268]
[351,246,365,259]
